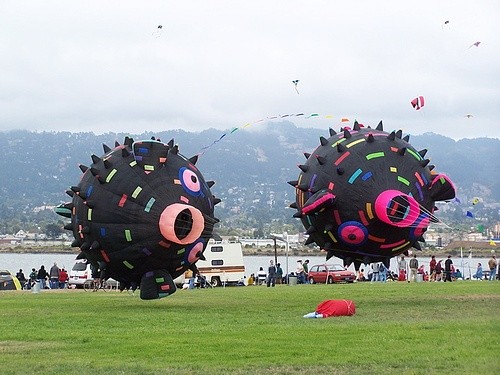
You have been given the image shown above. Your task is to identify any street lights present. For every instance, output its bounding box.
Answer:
[283,231,288,286]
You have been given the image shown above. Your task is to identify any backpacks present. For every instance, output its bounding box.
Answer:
[31,273,36,279]
[16,273,23,281]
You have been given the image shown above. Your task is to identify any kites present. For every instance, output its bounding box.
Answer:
[441,19,450,31]
[157,24,163,32]
[56,134,221,300]
[468,42,481,49]
[292,80,301,93]
[287,120,456,271]
[412,96,425,111]
[472,197,481,206]
[464,113,474,120]
[464,210,474,218]
[200,112,349,150]
[454,198,460,203]
[488,239,497,246]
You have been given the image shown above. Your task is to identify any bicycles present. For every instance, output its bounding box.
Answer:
[84,274,112,292]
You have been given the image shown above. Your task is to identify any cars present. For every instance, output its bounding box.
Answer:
[307,265,355,284]
[464,271,499,280]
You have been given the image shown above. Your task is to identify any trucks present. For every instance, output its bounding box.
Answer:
[173,243,246,287]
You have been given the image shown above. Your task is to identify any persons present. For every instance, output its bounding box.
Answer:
[284,259,309,285]
[92,277,100,292]
[257,267,267,284]
[38,265,50,281]
[418,265,430,282]
[487,254,498,280]
[398,256,407,280]
[188,274,206,289]
[356,262,398,284]
[29,267,36,281]
[472,263,483,281]
[238,273,256,285]
[266,260,278,288]
[408,254,418,283]
[58,268,69,289]
[49,262,60,288]
[429,255,463,282]
[14,269,27,290]
[276,263,282,284]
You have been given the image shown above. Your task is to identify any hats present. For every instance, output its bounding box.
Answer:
[277,263,281,265]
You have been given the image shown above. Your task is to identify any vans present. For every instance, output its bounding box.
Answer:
[69,260,119,287]
